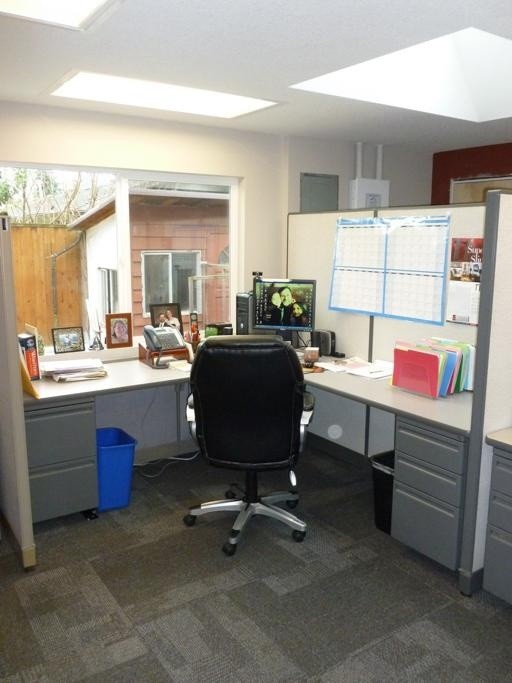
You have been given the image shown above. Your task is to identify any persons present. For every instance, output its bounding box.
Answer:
[291,299,311,326]
[165,307,181,330]
[112,320,130,344]
[64,332,74,346]
[155,313,172,327]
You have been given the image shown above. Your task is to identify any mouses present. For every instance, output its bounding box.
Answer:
[304,359,314,368]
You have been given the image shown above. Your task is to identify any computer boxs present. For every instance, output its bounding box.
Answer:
[235,290,277,334]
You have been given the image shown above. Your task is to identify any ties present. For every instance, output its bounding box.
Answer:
[161,324,162,326]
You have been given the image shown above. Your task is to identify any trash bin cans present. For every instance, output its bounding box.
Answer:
[369,449,395,536]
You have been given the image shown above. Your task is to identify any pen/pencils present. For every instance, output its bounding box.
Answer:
[185,324,199,342]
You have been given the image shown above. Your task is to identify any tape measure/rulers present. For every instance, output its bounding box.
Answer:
[190,313,198,329]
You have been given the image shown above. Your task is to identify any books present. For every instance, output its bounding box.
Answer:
[17,331,41,381]
[39,359,107,382]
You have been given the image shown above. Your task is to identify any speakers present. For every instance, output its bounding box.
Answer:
[311,329,319,348]
[319,330,336,356]
[190,313,198,331]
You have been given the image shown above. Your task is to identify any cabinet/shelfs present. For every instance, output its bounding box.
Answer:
[24,402,99,524]
[391,420,465,571]
[483,454,512,605]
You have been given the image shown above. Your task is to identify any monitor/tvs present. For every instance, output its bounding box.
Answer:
[252,278,317,351]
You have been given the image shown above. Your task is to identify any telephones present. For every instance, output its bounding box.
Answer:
[144,325,187,352]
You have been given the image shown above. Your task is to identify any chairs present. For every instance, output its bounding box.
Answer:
[183,335,315,556]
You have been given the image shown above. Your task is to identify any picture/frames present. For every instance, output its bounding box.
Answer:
[448,174,512,204]
[106,313,132,348]
[51,327,84,354]
[149,303,184,339]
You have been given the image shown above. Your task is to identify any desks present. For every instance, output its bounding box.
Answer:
[23,346,473,438]
